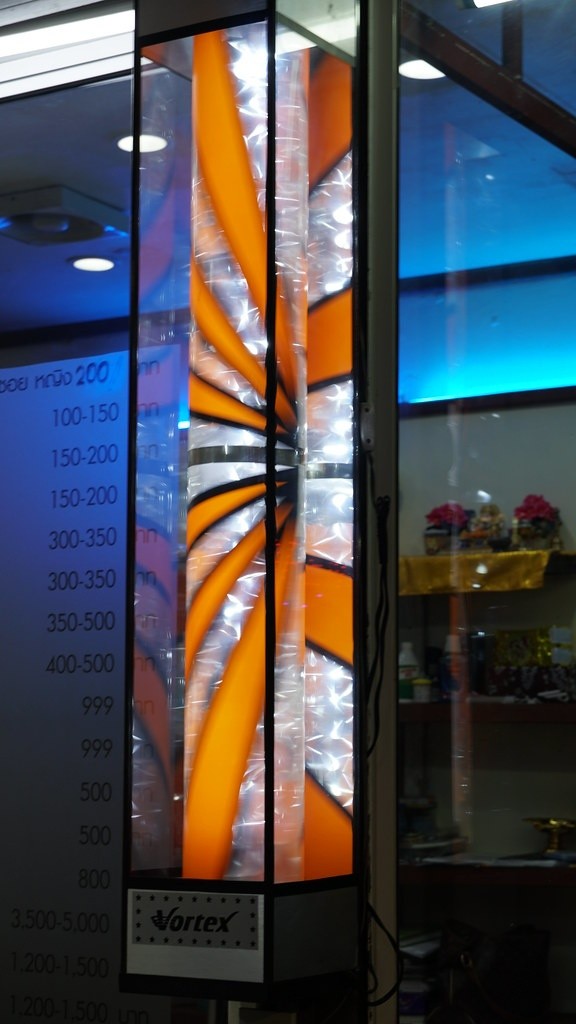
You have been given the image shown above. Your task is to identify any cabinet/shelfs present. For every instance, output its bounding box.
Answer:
[394,547,576,891]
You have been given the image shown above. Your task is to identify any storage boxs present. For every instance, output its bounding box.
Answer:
[467,625,575,700]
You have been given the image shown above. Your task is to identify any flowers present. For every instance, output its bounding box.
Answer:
[512,495,558,523]
[425,502,471,528]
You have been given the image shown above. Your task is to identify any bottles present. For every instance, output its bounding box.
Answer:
[396,640,421,704]
[412,678,432,703]
